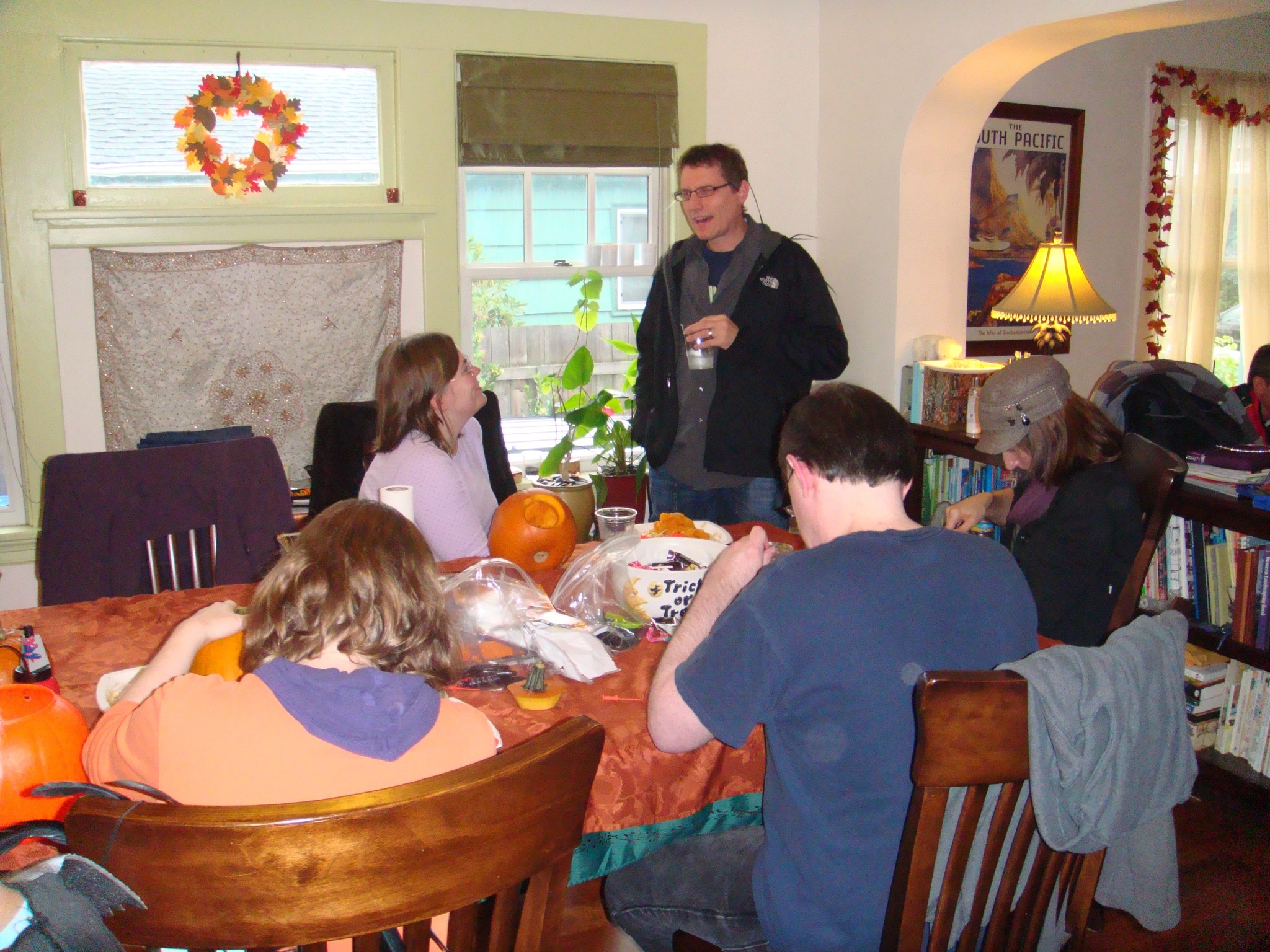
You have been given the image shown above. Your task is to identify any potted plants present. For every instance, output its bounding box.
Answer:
[532,270,648,545]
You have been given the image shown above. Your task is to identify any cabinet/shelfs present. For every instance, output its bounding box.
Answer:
[904,420,1270,809]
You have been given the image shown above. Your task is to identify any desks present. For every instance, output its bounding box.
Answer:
[0,519,813,952]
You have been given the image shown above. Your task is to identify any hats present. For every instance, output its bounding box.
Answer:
[973,355,1070,454]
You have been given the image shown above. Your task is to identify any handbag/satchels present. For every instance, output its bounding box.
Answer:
[1122,374,1246,459]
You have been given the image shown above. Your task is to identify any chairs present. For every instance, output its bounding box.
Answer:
[1100,432,1188,641]
[308,390,517,523]
[879,610,1198,952]
[64,716,606,951]
[40,436,297,607]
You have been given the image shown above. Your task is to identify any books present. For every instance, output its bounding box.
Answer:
[905,367,1270,788]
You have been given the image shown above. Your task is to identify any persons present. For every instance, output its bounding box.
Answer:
[80,490,504,952]
[1228,339,1270,448]
[934,354,1146,657]
[357,333,503,562]
[633,142,849,539]
[596,379,1063,952]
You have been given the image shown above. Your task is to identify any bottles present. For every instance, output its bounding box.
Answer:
[967,378,983,436]
[14,624,59,696]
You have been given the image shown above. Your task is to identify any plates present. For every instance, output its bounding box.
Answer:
[635,520,734,545]
[919,358,1006,375]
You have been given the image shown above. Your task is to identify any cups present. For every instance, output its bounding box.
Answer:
[595,507,638,543]
[681,323,715,369]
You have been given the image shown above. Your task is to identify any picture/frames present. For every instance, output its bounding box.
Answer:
[966,102,1085,357]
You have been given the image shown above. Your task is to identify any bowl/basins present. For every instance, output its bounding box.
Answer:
[95,666,149,713]
[611,537,729,622]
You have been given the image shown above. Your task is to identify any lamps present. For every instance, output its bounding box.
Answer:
[991,227,1117,356]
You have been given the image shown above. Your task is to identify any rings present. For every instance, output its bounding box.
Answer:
[709,329,713,338]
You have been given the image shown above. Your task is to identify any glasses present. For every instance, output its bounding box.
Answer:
[674,183,732,202]
